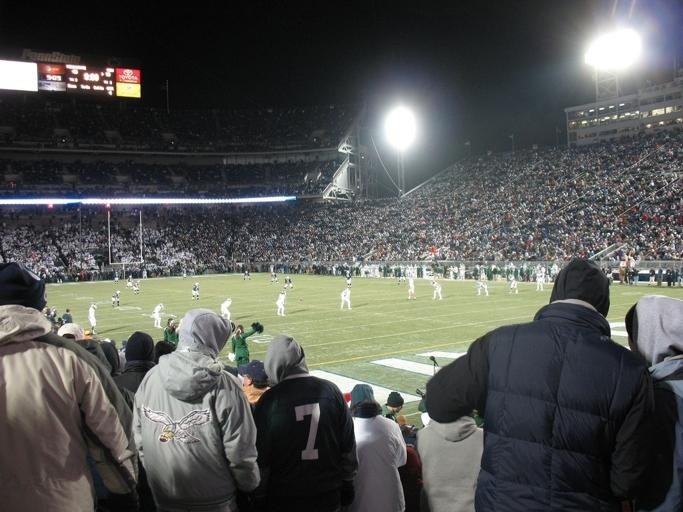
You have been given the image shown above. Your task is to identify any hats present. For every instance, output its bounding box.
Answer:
[125,331,155,362]
[57,322,86,339]
[0,260,46,311]
[386,389,405,408]
[238,358,268,382]
